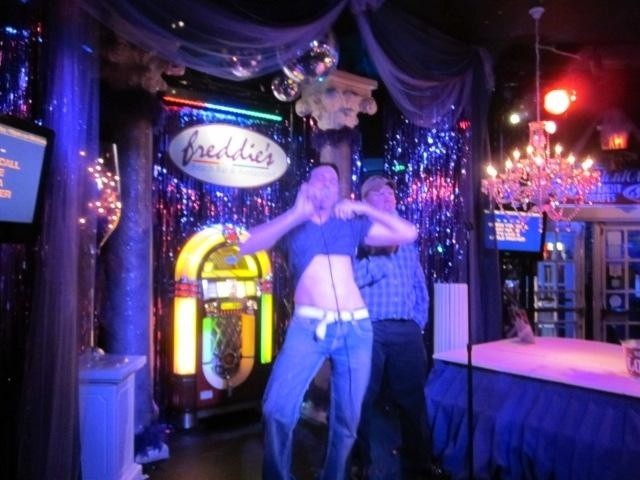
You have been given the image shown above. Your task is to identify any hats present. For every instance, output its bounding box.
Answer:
[360,175,398,197]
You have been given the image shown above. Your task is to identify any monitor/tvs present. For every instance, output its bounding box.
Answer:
[0,112,56,235]
[480,206,547,259]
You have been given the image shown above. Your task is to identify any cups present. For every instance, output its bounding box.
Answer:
[622,339,640,377]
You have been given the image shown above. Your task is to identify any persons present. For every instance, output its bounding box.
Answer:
[349,174,445,480]
[237,161,420,480]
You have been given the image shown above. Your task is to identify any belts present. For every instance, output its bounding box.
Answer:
[293,304,370,341]
